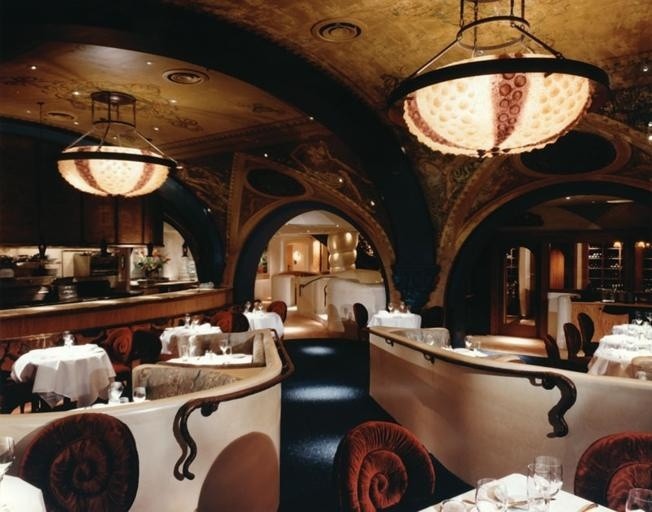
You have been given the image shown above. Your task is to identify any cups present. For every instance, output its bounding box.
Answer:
[408,329,484,351]
[106,381,148,405]
[0,436,15,482]
[524,453,563,512]
[438,497,465,511]
[624,488,652,512]
[471,477,509,512]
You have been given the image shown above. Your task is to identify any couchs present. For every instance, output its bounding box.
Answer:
[1,327,296,512]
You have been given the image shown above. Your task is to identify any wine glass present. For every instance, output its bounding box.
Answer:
[62,331,74,351]
[243,301,265,316]
[185,313,200,330]
[218,331,230,358]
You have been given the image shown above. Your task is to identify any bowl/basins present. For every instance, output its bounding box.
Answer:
[615,291,635,303]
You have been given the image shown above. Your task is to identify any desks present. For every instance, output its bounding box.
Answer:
[158,324,222,355]
[242,312,283,337]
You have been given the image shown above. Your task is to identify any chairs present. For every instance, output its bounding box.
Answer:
[265,302,288,326]
[353,303,369,345]
[572,429,652,512]
[210,310,245,334]
[328,420,442,512]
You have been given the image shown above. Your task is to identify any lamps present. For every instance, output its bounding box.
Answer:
[57,90,181,199]
[390,1,612,161]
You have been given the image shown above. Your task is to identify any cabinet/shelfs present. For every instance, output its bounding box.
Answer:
[143,196,164,246]
[638,246,652,291]
[582,242,623,289]
[82,196,143,245]
[0,131,82,245]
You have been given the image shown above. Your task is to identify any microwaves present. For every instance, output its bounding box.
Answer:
[74,253,119,278]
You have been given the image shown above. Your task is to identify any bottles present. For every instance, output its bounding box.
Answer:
[588,243,625,290]
